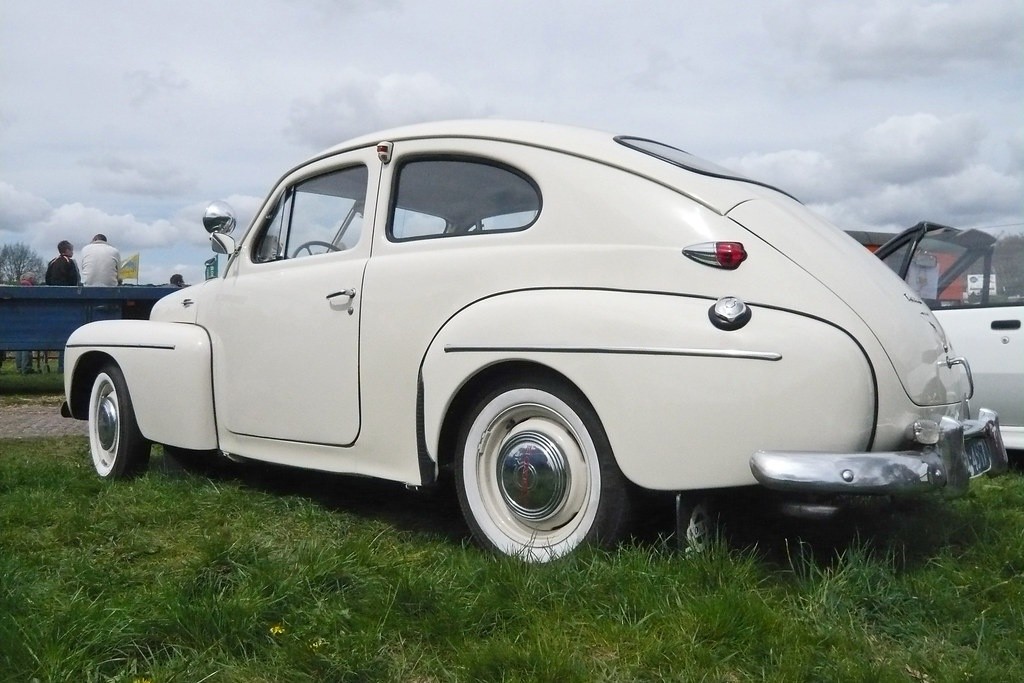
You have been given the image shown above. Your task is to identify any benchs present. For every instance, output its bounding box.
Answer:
[0,285,187,374]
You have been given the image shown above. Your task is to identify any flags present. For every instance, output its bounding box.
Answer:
[205,254,218,280]
[118,255,138,279]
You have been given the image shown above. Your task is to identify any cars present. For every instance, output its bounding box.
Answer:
[872,219,1024,453]
[60,118,1008,570]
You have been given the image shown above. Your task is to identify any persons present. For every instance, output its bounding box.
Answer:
[13,271,38,375]
[170,274,185,285]
[45,240,82,286]
[80,234,123,287]
